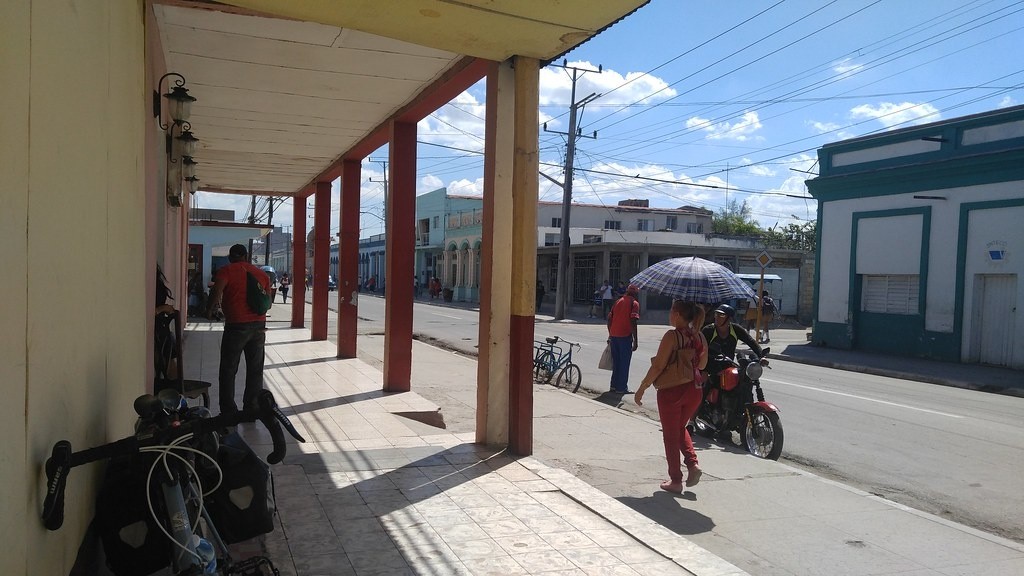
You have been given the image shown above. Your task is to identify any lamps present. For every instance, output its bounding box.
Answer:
[177,155,198,178]
[166,119,199,163]
[152,73,196,130]
[183,174,200,195]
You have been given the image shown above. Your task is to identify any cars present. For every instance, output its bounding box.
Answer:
[328,275,337,291]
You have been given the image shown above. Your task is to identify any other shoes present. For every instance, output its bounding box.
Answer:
[685,466,702,487]
[610,386,634,394]
[588,313,596,318]
[660,477,683,494]
[604,317,606,320]
[764,339,770,343]
[686,425,694,436]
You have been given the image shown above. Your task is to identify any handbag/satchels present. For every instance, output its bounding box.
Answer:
[192,432,276,545]
[279,286,283,291]
[242,266,270,315]
[650,326,694,389]
[597,342,614,370]
[95,452,172,576]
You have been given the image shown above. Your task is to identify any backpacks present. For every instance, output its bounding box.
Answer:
[762,297,774,315]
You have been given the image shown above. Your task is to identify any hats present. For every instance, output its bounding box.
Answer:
[230,244,248,258]
[627,285,640,293]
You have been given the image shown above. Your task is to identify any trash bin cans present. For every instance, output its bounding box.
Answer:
[271,287,278,303]
[443,288,454,303]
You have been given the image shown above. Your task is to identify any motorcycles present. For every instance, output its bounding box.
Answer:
[693,347,783,461]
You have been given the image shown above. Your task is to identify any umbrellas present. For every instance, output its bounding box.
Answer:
[629,253,756,304]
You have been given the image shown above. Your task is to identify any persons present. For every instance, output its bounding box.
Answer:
[305,277,309,292]
[743,288,780,345]
[635,299,708,494]
[686,304,769,449]
[536,280,545,312]
[358,275,443,300]
[587,280,627,320]
[607,285,640,394]
[280,273,290,304]
[215,244,272,417]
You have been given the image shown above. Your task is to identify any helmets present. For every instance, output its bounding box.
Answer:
[714,303,735,318]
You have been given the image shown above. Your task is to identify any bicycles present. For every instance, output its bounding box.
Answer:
[536,337,581,393]
[41,387,305,576]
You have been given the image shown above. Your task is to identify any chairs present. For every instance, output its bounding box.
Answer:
[154,310,212,411]
